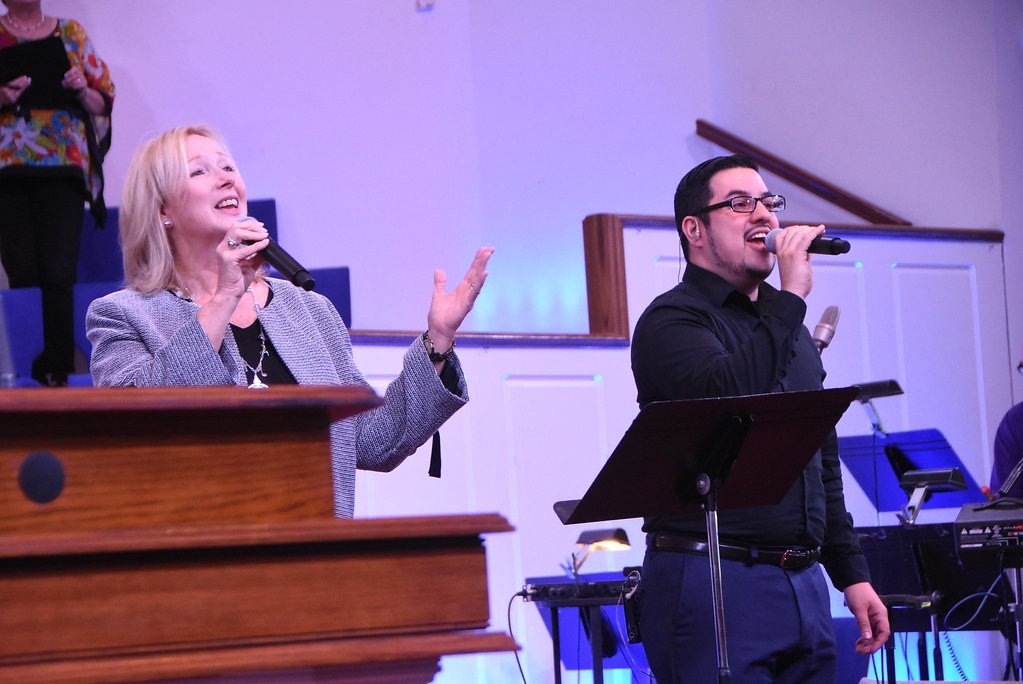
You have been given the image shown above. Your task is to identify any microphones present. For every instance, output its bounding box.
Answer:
[765,228,850,255]
[238,216,315,292]
[812,304,840,354]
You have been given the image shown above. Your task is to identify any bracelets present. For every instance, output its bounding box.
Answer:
[79,88,88,101]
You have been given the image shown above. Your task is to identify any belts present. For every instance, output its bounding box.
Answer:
[646,534,821,572]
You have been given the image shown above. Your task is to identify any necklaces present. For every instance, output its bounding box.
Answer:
[6,12,44,30]
[241,285,269,385]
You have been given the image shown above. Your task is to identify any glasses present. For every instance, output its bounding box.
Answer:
[679,194,786,239]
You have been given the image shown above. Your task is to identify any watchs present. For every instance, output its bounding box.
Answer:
[422,330,456,362]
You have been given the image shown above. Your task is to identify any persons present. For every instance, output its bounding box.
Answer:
[0,0,116,385]
[87,124,495,519]
[631,155,889,684]
[988,342,1023,498]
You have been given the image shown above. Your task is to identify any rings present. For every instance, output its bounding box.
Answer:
[77,78,80,81]
[228,237,239,249]
[470,284,481,294]
